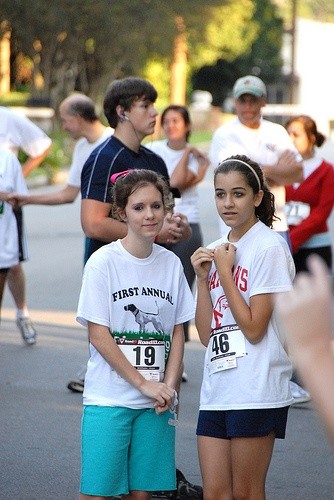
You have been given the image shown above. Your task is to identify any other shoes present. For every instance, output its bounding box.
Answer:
[67,378,85,392]
[17,314,37,345]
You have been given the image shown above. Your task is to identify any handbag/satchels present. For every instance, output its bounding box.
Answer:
[151,467,204,500]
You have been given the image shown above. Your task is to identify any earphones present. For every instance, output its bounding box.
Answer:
[120,111,130,121]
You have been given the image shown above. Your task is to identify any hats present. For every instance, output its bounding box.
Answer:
[232,74,267,99]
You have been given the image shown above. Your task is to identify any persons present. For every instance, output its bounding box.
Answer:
[0,191,17,210]
[0,105,53,349]
[283,113,334,278]
[275,253,334,441]
[79,78,194,279]
[207,76,313,404]
[190,154,296,500]
[140,105,210,384]
[7,93,117,394]
[75,167,195,500]
